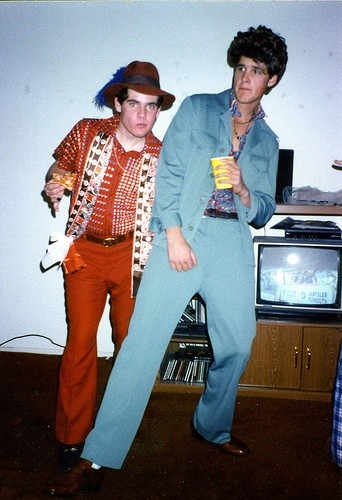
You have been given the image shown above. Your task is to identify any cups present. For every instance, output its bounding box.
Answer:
[211,156,234,189]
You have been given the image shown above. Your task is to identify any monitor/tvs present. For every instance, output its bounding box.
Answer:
[251,235,342,314]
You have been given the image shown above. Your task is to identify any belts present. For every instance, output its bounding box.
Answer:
[204,209,238,219]
[80,231,133,246]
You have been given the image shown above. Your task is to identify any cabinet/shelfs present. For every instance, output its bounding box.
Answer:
[151,203,342,399]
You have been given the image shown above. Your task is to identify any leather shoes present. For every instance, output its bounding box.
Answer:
[192,427,249,455]
[45,459,103,496]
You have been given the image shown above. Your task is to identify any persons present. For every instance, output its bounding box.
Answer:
[41,25,288,498]
[41,60,174,474]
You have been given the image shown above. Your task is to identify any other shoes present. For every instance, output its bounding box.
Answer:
[57,443,84,472]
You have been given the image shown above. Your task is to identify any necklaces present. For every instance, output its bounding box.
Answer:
[113,148,142,173]
[233,105,263,139]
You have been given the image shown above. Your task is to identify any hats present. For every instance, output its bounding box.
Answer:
[95,61,175,110]
